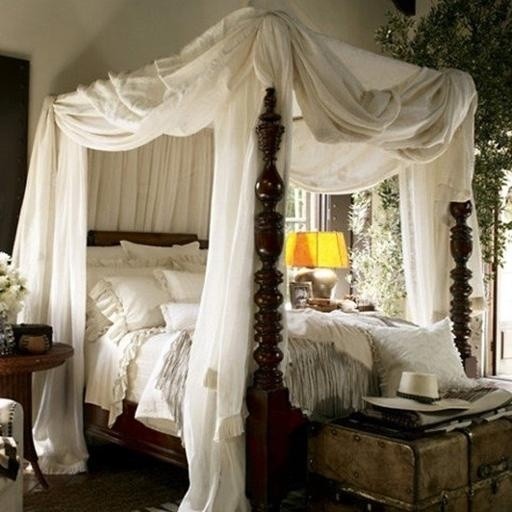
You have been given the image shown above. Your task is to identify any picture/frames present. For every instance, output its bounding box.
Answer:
[290,281,313,309]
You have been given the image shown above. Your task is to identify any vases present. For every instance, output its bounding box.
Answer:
[0,322,15,355]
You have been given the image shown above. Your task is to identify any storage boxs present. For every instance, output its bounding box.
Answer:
[310,406,511,511]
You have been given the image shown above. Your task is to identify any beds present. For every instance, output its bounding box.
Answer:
[15,1,489,512]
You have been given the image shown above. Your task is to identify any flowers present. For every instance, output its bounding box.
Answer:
[0,253,28,320]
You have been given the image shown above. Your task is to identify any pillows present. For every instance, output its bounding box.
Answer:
[85,238,211,337]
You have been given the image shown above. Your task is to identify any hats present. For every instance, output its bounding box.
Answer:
[362,371,471,412]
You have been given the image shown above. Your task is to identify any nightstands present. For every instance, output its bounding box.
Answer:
[306,303,373,314]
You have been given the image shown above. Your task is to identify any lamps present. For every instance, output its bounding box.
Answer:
[290,229,349,301]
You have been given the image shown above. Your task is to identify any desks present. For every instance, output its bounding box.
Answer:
[1,341,75,493]
